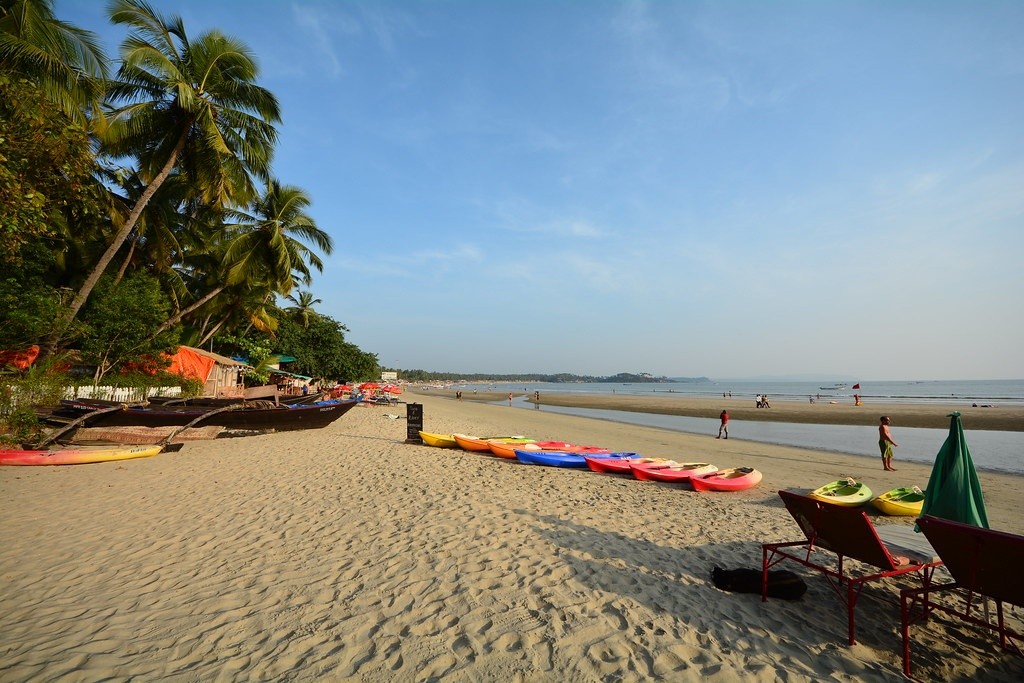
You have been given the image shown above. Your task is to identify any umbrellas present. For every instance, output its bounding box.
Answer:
[329,383,402,396]
[913,410,994,635]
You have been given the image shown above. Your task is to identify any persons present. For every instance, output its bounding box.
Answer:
[456,388,540,403]
[323,394,332,411]
[302,383,310,396]
[722,390,771,408]
[878,416,898,471]
[714,410,729,439]
[809,394,860,405]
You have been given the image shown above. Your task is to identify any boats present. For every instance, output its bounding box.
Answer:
[418,431,607,459]
[820,386,847,390]
[0,443,165,466]
[687,466,762,492]
[584,456,677,472]
[629,461,718,482]
[30,392,365,431]
[870,485,927,515]
[513,448,642,467]
[807,477,873,506]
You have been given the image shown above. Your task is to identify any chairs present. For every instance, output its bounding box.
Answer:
[762,491,1024,678]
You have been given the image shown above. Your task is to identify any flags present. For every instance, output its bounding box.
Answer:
[852,384,860,390]
